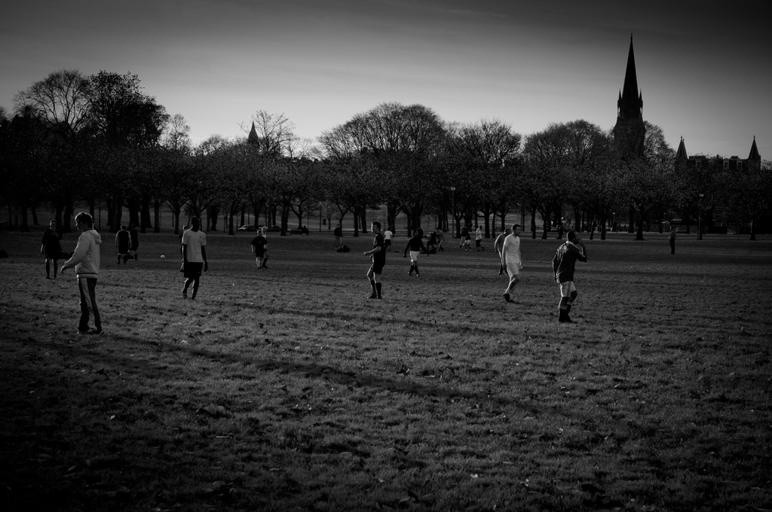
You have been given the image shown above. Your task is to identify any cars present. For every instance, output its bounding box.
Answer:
[238,223,281,232]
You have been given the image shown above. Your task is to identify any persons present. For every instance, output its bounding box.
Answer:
[61,212,103,334]
[552,231,587,322]
[384,227,393,251]
[667,225,675,255]
[250,230,267,270]
[128,224,140,262]
[39,216,61,281]
[501,224,523,302]
[182,216,209,300]
[403,229,428,278]
[427,225,482,256]
[364,221,386,300]
[114,225,132,263]
[494,229,510,277]
[334,223,343,249]
[179,225,190,272]
[261,226,270,268]
